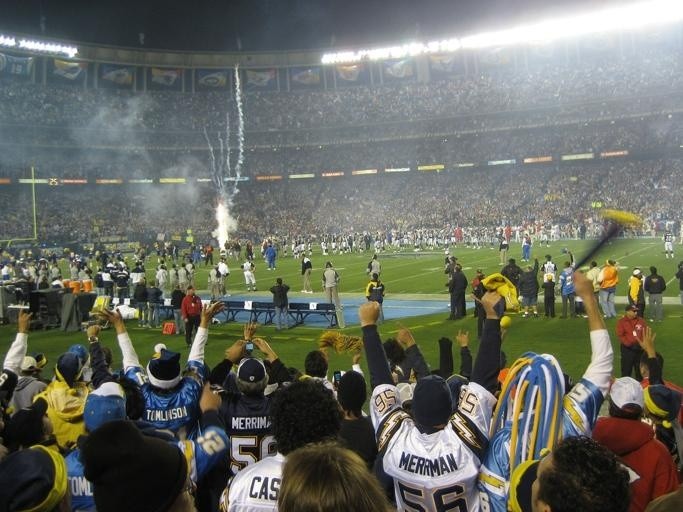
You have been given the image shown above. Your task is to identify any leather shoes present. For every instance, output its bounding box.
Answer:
[446,312,464,321]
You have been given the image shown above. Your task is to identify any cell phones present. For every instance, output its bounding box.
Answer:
[334,370,341,388]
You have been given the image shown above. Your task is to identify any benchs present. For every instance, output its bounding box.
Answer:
[287,302,337,328]
[223,301,277,323]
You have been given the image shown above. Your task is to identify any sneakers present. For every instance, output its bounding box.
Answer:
[522,312,666,323]
[301,288,314,295]
[247,285,258,292]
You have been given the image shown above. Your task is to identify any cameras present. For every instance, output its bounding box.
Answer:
[246,343,254,352]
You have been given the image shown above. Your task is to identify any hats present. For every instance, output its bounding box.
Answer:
[83,380,127,435]
[21,343,91,395]
[411,375,452,428]
[236,356,268,385]
[372,254,378,259]
[372,272,379,278]
[608,376,646,411]
[443,250,570,285]
[146,343,183,390]
[507,447,552,512]
[0,369,48,447]
[78,418,188,512]
[633,269,642,276]
[0,444,68,512]
[1,252,146,275]
[644,384,683,430]
[624,304,640,312]
[325,260,333,268]
